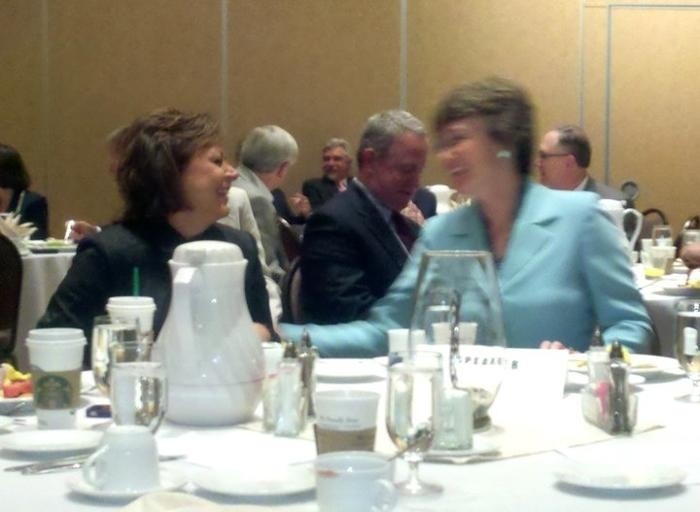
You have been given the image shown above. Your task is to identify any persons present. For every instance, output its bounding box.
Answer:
[271,137,358,226]
[301,109,429,323]
[682,245,700,272]
[402,184,437,227]
[39,103,282,371]
[228,124,298,272]
[536,125,629,201]
[279,76,658,358]
[0,142,47,245]
[64,125,131,241]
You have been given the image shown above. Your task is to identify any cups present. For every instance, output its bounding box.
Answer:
[679,229,700,245]
[652,224,672,238]
[312,452,402,512]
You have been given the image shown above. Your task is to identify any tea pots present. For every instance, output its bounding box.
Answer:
[150,240,267,427]
[595,196,646,253]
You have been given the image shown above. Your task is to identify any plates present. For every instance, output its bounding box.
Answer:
[0,213,81,257]
[0,287,694,492]
[65,470,186,502]
[183,447,314,499]
[623,238,700,297]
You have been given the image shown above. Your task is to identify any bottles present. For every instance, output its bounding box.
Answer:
[401,244,508,432]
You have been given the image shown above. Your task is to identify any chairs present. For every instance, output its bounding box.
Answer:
[277,214,303,325]
[639,209,700,258]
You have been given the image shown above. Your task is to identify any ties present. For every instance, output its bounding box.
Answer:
[391,211,417,253]
